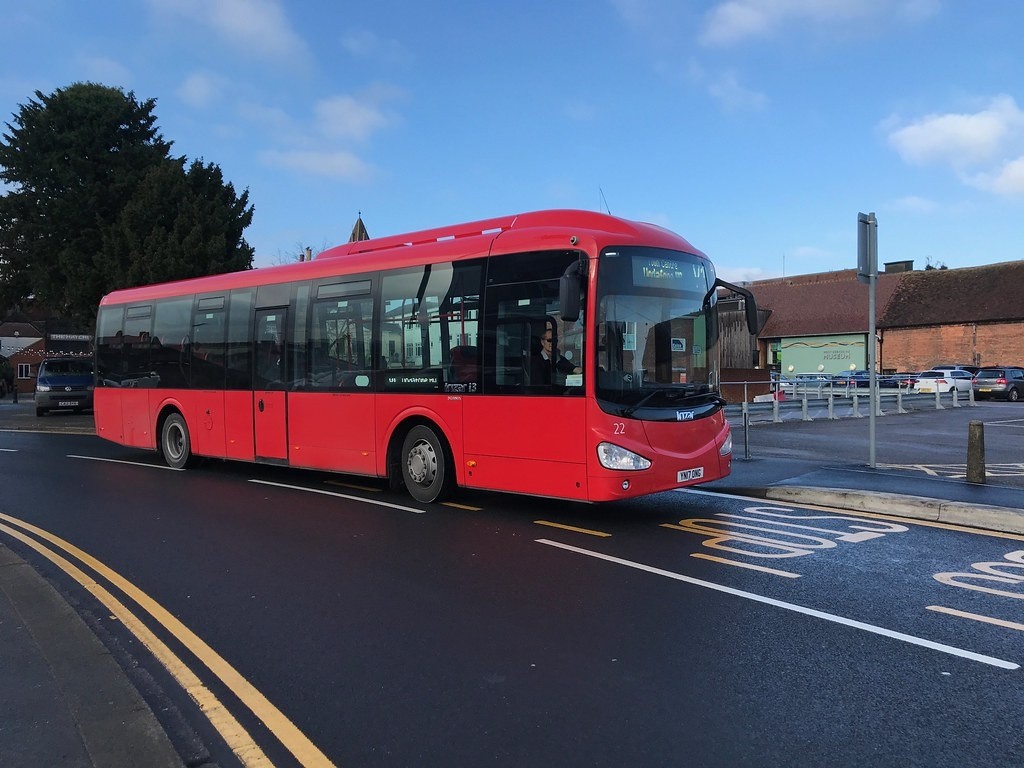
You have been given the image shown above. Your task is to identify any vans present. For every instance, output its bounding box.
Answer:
[28,357,94,417]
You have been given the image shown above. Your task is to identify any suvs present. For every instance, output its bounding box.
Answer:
[914,365,1024,401]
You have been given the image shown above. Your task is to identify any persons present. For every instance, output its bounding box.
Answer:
[524,328,606,396]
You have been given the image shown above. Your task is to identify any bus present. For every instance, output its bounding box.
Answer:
[93,209,759,504]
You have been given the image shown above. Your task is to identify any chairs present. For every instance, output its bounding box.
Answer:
[449,345,478,383]
[162,333,242,369]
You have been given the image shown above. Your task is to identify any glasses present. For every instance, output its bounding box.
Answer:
[544,339,558,342]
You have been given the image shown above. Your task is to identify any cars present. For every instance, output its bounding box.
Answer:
[770,370,921,397]
